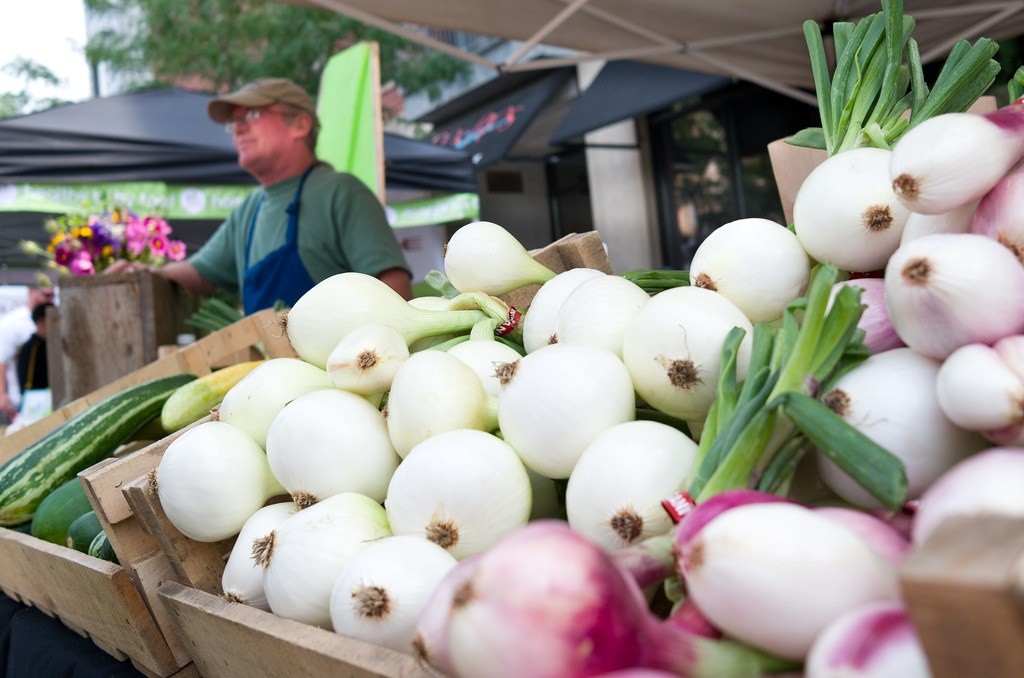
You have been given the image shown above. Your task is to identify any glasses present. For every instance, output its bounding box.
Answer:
[224,108,296,131]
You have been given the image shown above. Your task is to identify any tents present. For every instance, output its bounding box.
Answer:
[0,84,474,267]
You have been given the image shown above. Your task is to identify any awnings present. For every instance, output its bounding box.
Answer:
[547,59,729,148]
[419,69,575,166]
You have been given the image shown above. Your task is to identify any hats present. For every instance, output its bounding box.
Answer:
[209,77,317,123]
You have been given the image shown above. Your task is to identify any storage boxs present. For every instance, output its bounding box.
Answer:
[0,247,542,678]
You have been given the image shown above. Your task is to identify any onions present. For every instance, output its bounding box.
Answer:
[151,109,1024,678]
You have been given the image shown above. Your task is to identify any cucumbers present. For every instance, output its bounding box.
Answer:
[0,361,261,567]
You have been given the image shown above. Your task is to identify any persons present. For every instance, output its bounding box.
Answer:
[105,75,413,318]
[0,284,60,432]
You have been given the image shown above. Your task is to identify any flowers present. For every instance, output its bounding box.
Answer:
[16,189,187,289]
